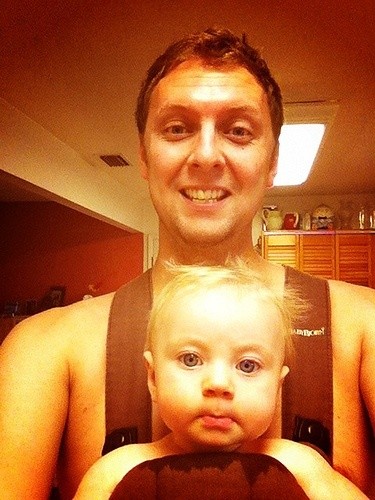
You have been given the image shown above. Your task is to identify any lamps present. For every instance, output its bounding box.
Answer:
[273,100,341,187]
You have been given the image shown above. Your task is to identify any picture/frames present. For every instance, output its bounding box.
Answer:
[46,285,66,307]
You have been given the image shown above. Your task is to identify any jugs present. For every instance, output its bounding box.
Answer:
[261,209,283,230]
[283,212,300,229]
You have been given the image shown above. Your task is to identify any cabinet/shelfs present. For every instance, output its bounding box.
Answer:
[261,229,375,290]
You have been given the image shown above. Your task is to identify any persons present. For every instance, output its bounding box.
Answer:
[71,253,372,499]
[1,25,375,499]
